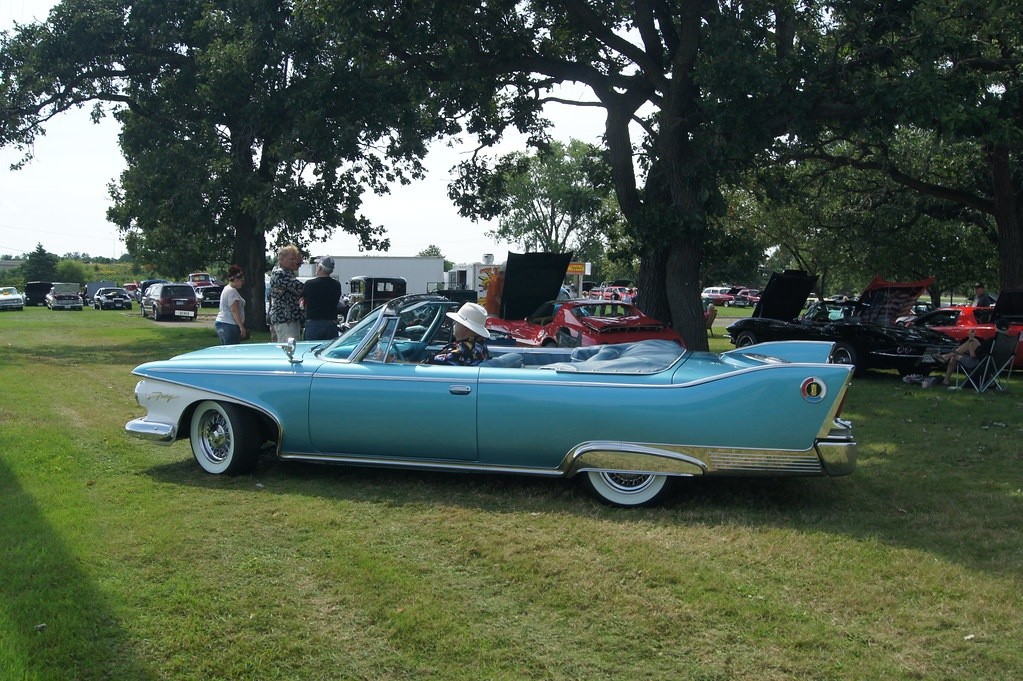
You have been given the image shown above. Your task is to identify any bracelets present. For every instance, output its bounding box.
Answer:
[390,354,398,364]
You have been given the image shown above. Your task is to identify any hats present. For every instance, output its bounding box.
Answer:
[319,256,335,269]
[994,318,1009,332]
[445,302,491,339]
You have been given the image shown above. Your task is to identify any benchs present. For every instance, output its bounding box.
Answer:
[536,345,622,371]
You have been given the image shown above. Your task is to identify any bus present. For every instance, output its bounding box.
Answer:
[187,272,224,293]
[122,283,141,300]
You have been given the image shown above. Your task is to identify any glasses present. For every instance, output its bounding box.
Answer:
[291,260,305,266]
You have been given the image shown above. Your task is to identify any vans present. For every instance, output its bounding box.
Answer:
[139,282,199,322]
[21,281,54,307]
[700,287,732,299]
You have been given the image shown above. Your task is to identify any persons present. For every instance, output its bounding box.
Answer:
[970,282,993,326]
[299,255,343,341]
[214,265,247,345]
[373,301,492,365]
[268,246,307,342]
[931,317,1011,386]
[839,296,860,320]
[580,289,637,317]
[704,303,714,319]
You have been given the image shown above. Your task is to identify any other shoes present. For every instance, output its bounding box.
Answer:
[930,352,948,365]
[936,379,950,386]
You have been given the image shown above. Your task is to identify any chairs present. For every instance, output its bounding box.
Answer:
[705,309,718,337]
[476,351,526,369]
[954,330,1022,395]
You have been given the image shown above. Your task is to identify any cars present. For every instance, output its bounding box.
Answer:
[705,285,749,308]
[0,286,24,310]
[341,275,417,325]
[133,280,168,305]
[906,287,1023,373]
[721,275,962,386]
[485,298,687,352]
[122,250,861,510]
[829,295,850,303]
[894,301,937,326]
[93,286,132,310]
[193,285,222,309]
[737,289,763,306]
[45,283,83,312]
[957,292,999,309]
[551,287,589,327]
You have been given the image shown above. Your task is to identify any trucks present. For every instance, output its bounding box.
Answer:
[265,255,447,325]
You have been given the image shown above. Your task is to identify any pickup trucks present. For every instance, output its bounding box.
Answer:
[589,286,636,303]
[803,292,836,309]
